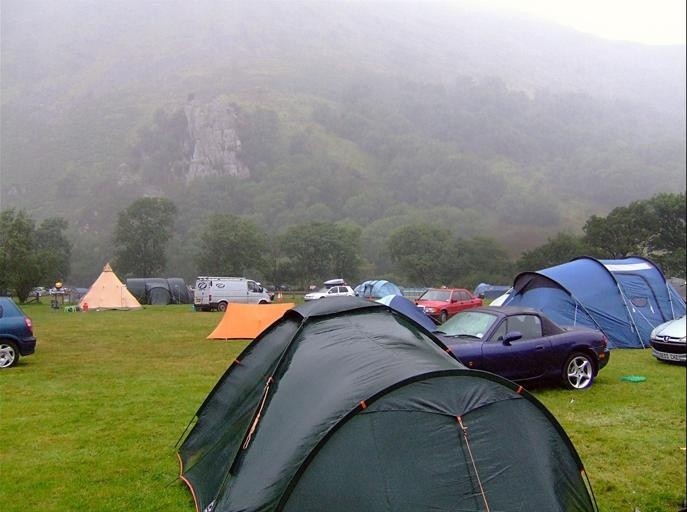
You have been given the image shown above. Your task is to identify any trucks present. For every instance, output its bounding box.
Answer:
[194,276,270,312]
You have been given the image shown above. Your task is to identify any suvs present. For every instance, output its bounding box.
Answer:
[303,279,355,301]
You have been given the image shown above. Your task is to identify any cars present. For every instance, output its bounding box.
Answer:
[488,286,541,323]
[0,297,36,368]
[414,289,482,325]
[649,313,687,364]
[431,308,610,389]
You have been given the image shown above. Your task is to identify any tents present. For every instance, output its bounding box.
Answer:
[175,295,599,512]
[81,262,143,309]
[354,279,405,297]
[474,282,512,298]
[503,256,686,349]
[207,303,294,340]
[374,294,440,333]
